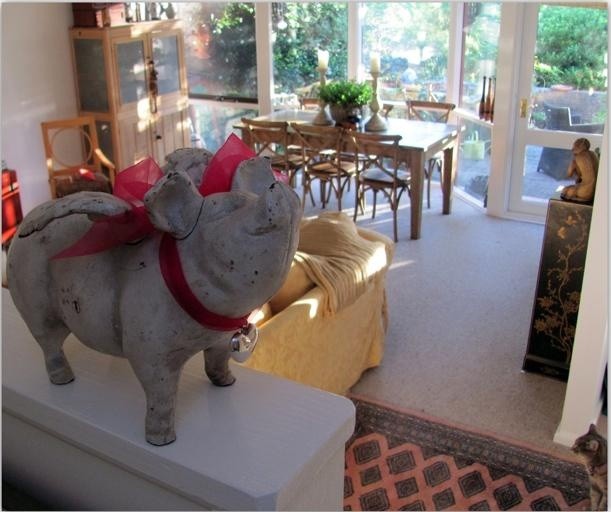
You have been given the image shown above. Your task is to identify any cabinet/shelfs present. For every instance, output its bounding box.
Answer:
[71,19,191,177]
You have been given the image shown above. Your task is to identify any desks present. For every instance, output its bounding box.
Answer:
[234,109,466,239]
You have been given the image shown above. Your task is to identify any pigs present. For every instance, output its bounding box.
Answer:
[5,147,304,447]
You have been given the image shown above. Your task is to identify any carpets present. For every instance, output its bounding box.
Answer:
[345,394,592,512]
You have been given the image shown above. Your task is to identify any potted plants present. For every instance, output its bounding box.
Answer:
[315,80,374,126]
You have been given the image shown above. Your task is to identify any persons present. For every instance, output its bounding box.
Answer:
[396,57,419,85]
[563,137,599,200]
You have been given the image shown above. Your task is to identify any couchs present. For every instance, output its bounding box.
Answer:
[229,213,397,396]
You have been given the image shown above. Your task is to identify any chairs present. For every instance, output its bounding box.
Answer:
[41,115,116,201]
[240,97,457,245]
[536,101,605,182]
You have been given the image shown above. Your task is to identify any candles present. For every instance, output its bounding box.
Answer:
[318,51,330,69]
[372,53,381,72]
